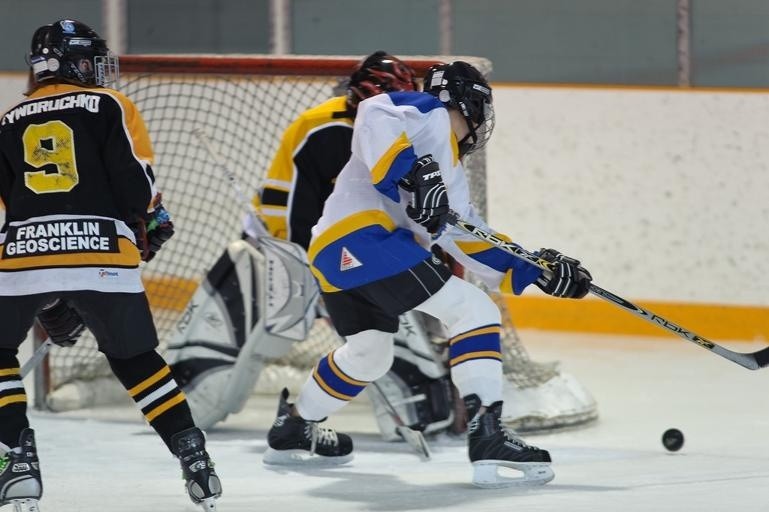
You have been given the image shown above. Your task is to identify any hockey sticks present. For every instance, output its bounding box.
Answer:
[192,125,431,463]
[446,206,769,370]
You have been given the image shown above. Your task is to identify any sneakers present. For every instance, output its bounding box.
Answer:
[461,394,552,462]
[0,430,41,504]
[170,428,222,504]
[268,387,352,456]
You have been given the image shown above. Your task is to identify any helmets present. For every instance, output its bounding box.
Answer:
[424,61,495,159]
[25,19,120,92]
[345,51,419,121]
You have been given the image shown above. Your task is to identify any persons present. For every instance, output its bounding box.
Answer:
[0,16,223,506]
[160,48,455,445]
[265,60,594,465]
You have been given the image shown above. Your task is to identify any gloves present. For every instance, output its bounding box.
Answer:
[400,154,449,232]
[136,205,175,262]
[532,248,592,298]
[34,300,86,347]
